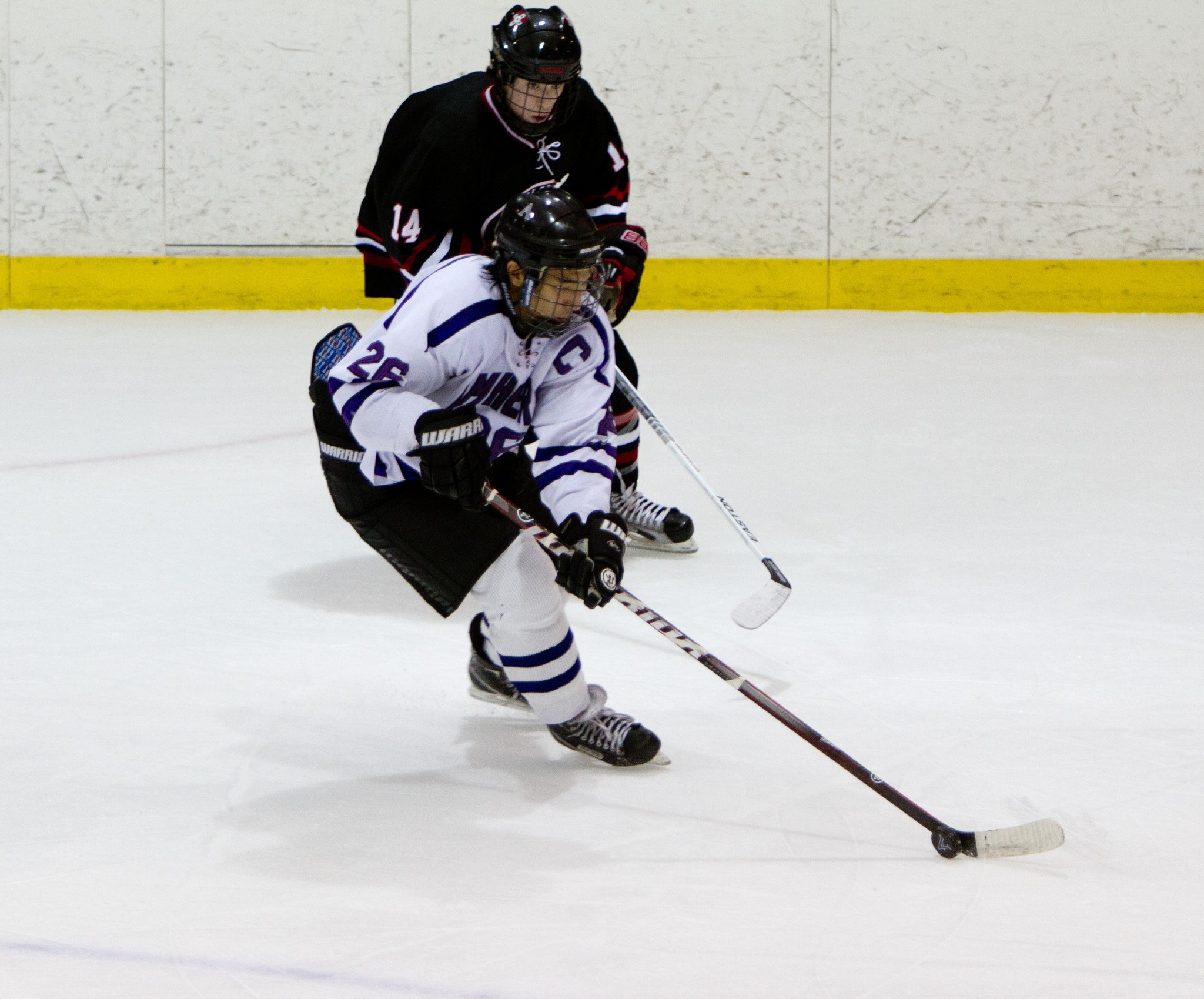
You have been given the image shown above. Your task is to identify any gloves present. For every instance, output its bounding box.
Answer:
[414,402,490,511]
[550,510,628,608]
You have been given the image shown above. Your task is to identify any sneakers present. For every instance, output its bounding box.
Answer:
[608,484,699,552]
[545,704,673,768]
[466,611,544,713]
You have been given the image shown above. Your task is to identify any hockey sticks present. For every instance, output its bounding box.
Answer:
[477,477,1064,858]
[611,363,792,630]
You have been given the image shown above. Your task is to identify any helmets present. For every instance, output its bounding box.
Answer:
[495,186,603,338]
[489,5,582,137]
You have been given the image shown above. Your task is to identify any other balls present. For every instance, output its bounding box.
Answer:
[931,826,963,858]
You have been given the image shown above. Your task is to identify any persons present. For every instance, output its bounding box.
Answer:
[355,4,696,554]
[310,187,670,767]
